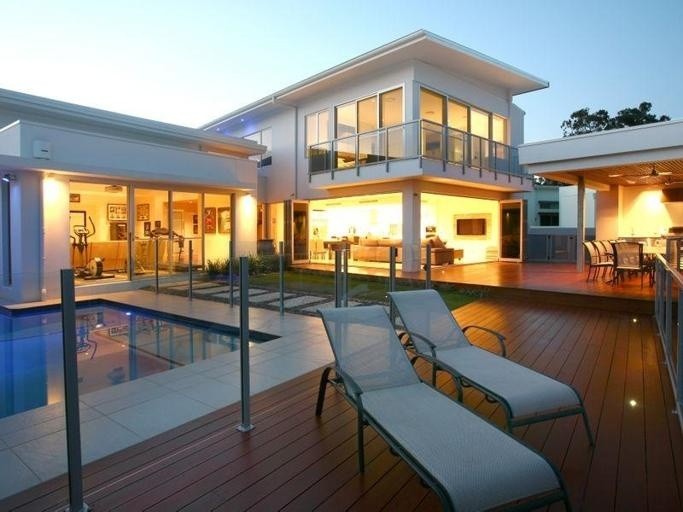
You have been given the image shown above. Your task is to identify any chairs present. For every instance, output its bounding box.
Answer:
[581,239,656,289]
[315,304,571,508]
[383,289,593,458]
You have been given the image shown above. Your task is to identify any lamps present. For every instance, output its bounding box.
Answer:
[105,185,122,193]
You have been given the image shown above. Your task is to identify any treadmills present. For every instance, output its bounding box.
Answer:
[149,228,203,272]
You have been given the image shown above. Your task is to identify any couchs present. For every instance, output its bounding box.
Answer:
[352,239,454,265]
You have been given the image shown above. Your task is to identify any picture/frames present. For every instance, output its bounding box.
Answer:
[108,203,149,221]
[205,207,230,233]
[70,194,80,202]
